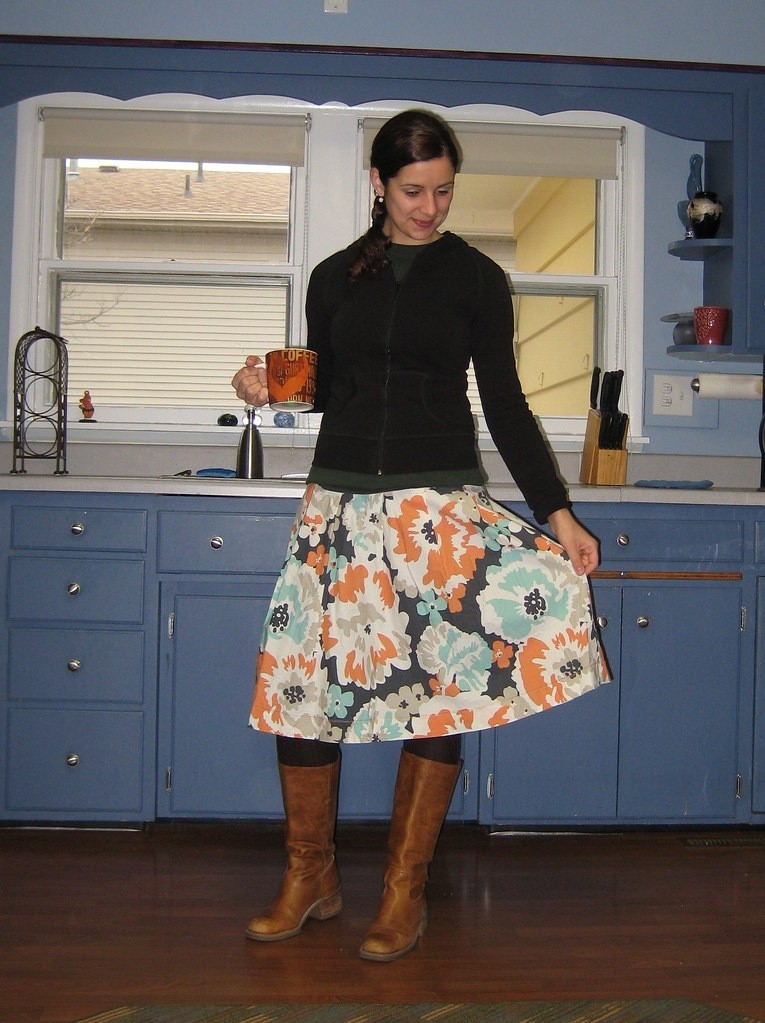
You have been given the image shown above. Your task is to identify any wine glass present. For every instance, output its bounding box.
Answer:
[677,201,695,240]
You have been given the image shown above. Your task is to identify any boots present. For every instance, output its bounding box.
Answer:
[359,748,463,961]
[246,749,344,940]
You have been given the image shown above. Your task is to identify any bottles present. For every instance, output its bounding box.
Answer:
[686,190,726,239]
[236,409,263,479]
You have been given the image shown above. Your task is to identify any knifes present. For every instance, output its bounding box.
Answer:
[577,366,632,485]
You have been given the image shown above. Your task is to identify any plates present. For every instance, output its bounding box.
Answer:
[273,411,295,428]
[197,468,236,478]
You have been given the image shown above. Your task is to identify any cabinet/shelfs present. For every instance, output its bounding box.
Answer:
[657,88,765,363]
[0,490,765,830]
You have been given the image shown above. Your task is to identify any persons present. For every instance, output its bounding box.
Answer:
[230,109,611,961]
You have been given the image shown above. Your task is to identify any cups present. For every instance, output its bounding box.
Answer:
[694,306,729,345]
[253,348,318,413]
